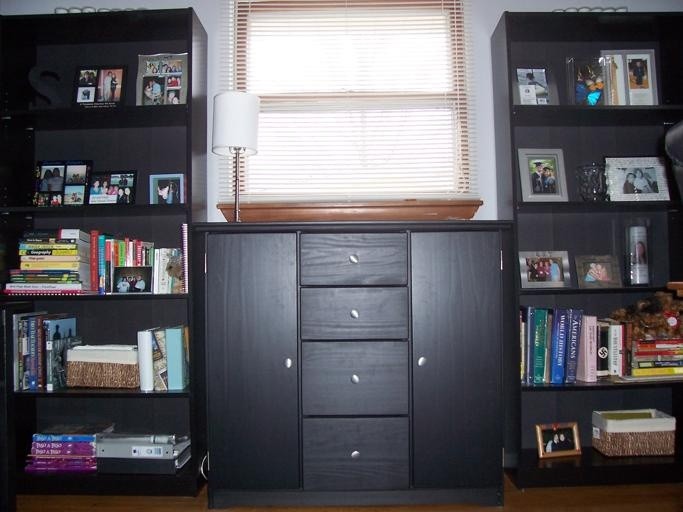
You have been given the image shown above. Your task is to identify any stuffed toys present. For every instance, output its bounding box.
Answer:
[607,291,682,340]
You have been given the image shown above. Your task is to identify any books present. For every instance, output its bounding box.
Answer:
[518,303,683,385]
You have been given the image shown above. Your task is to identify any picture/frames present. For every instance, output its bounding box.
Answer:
[602,156,674,204]
[135,53,188,105]
[534,422,583,460]
[515,146,568,204]
[28,160,92,207]
[148,173,184,206]
[512,48,660,107]
[72,64,126,107]
[572,254,623,288]
[87,171,137,208]
[517,249,572,288]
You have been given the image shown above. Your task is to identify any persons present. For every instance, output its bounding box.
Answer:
[623,172,641,194]
[527,241,651,288]
[558,432,570,451]
[34,167,179,207]
[525,71,546,93]
[632,168,655,194]
[540,165,556,195]
[631,60,647,87]
[76,59,181,106]
[545,433,561,454]
[531,161,545,195]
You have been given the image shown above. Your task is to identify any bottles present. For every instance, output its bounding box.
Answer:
[623,224,649,286]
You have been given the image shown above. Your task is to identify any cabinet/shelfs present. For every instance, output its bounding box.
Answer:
[490,5,679,489]
[2,8,208,500]
[194,220,514,506]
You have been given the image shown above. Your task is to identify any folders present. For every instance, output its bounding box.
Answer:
[96,436,190,459]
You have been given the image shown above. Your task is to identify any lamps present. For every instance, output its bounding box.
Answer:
[211,90,257,220]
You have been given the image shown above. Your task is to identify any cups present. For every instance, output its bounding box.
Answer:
[575,165,609,201]
